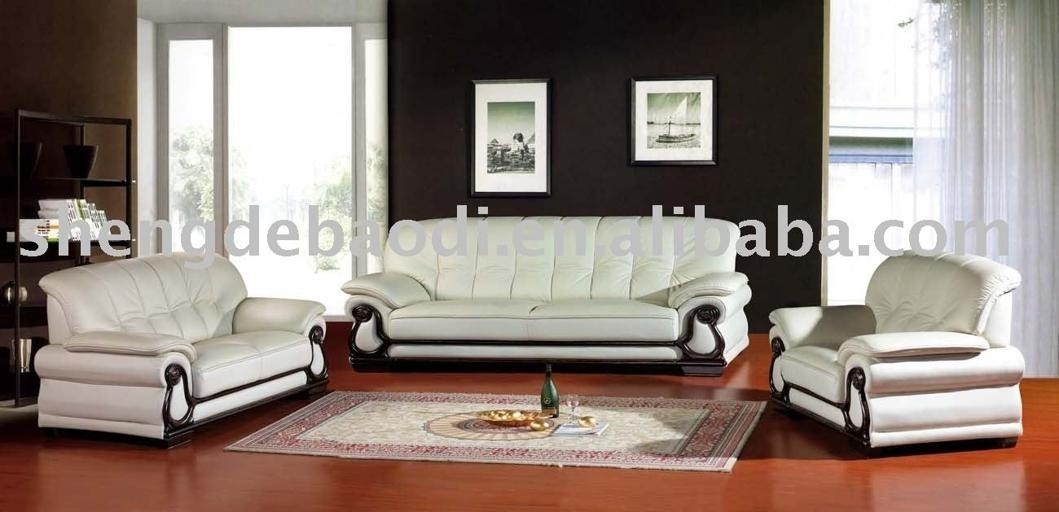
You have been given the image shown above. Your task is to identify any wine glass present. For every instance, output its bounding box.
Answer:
[567,393,579,422]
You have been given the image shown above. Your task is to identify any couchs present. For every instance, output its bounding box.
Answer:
[769,249,1026,456]
[34,250,329,451]
[341,217,752,378]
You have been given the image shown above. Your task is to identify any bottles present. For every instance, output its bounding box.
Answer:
[541,362,559,418]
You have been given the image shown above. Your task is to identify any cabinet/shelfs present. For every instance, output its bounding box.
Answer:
[0,109,136,409]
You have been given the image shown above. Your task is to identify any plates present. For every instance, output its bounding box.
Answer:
[477,409,553,426]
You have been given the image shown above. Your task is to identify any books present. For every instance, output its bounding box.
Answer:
[4,197,112,244]
[549,422,612,439]
[8,337,34,375]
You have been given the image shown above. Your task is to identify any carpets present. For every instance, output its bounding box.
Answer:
[224,390,769,473]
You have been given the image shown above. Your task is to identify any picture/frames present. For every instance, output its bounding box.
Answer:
[468,78,553,199]
[630,75,718,166]
